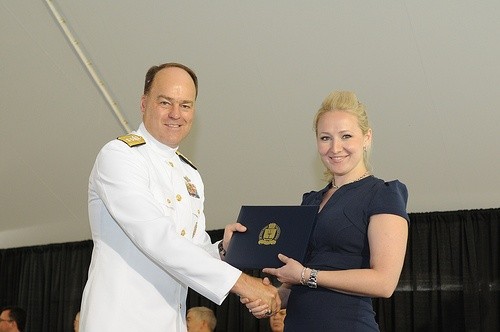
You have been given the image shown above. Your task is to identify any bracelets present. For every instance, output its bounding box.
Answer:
[300,267,309,286]
[218,241,226,257]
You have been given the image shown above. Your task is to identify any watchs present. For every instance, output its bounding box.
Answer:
[307,269,318,288]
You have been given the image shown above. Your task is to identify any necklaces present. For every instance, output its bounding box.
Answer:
[331,173,369,187]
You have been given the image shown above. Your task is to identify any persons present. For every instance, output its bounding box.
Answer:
[73,312,80,332]
[187,307,217,332]
[0,306,27,332]
[239,90,410,332]
[79,63,281,332]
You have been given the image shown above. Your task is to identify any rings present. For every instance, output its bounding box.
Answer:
[266,310,272,315]
